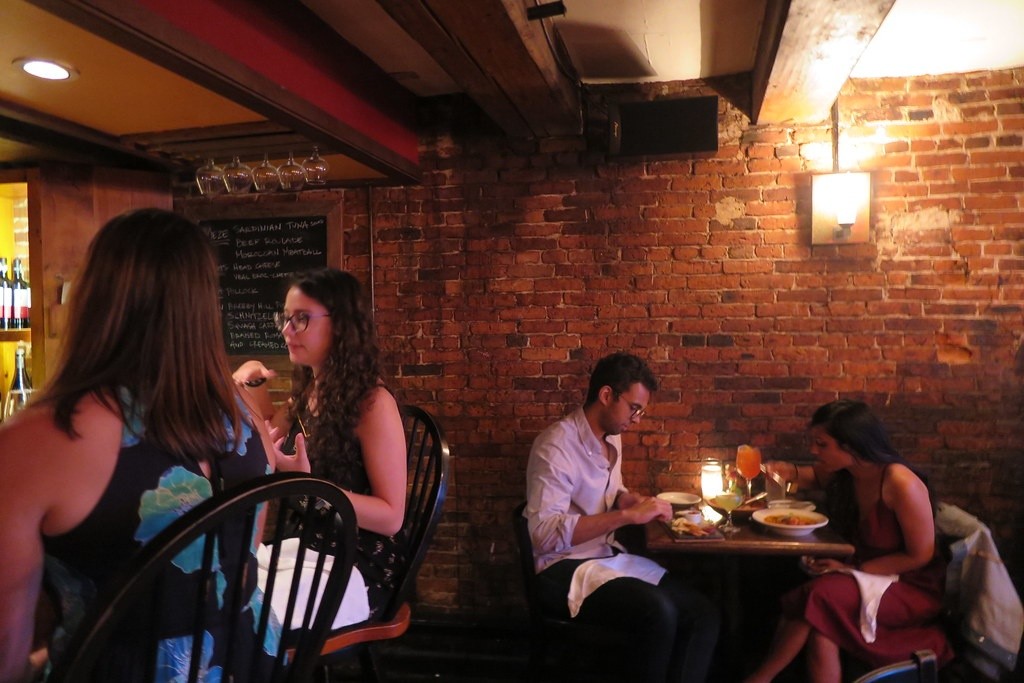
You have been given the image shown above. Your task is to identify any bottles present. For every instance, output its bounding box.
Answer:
[12,259,29,328]
[0,392,2,421]
[0,258,12,329]
[11,349,32,390]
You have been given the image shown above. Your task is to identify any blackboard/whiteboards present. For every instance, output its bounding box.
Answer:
[173,190,345,372]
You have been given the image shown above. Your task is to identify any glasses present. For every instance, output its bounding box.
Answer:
[274,311,331,332]
[611,389,648,419]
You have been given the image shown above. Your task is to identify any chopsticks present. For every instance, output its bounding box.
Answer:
[655,518,675,543]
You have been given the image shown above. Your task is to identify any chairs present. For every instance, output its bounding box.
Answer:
[511,500,655,683]
[46,470,358,683]
[281,405,450,683]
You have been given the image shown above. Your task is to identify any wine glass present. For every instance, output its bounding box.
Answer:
[736,445,761,499]
[267,369,305,456]
[714,479,748,532]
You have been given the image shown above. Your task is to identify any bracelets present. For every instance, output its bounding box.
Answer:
[794,464,798,479]
[244,377,266,387]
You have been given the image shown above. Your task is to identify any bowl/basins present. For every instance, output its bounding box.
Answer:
[769,500,816,512]
[709,496,767,521]
[752,509,829,537]
[685,511,701,524]
[657,492,701,509]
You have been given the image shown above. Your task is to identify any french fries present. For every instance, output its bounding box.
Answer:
[671,518,693,535]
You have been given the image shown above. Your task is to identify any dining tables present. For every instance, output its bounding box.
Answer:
[646,502,855,556]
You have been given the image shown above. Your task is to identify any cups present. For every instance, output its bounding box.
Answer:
[4,391,32,422]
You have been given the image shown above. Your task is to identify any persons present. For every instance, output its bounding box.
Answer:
[523,350,721,683]
[741,398,947,683]
[232,266,407,649]
[0,207,288,683]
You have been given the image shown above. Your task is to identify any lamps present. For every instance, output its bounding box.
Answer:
[833,203,856,238]
